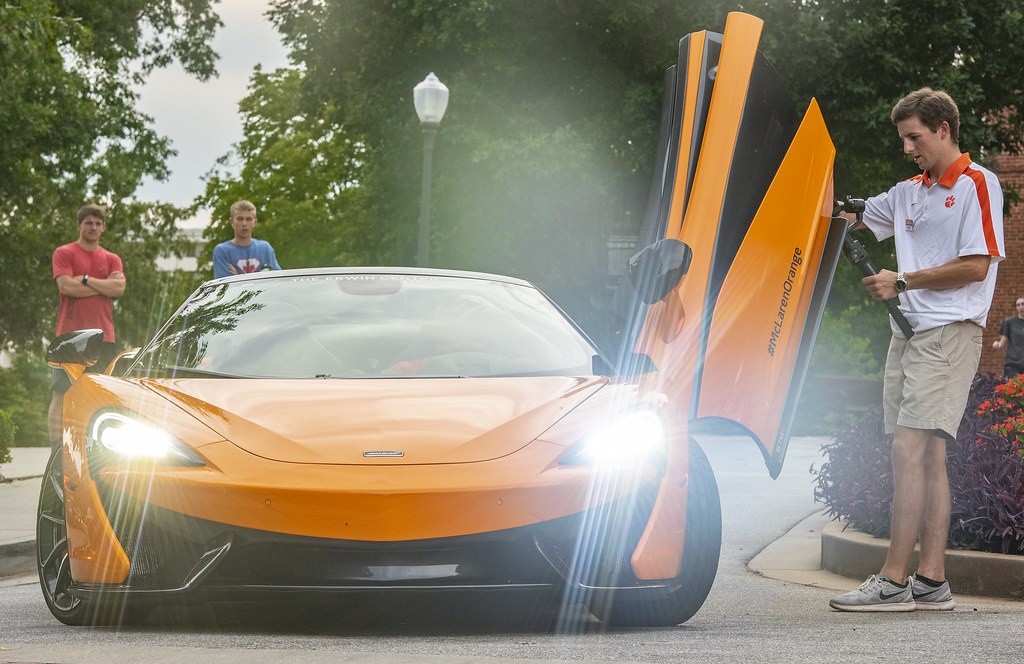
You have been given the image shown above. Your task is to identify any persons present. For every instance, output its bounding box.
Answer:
[213,200,283,279]
[47,202,126,450]
[993,296,1024,381]
[829,86,1008,612]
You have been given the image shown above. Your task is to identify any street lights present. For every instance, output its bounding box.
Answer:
[410,72,450,268]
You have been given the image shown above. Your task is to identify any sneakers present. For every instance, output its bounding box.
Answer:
[829,574,917,611]
[907,575,955,610]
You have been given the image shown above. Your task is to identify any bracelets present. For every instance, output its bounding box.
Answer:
[999,342,1002,349]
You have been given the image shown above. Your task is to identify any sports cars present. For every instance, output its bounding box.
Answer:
[33,12,844,637]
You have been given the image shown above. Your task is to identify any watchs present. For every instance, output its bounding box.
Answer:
[894,271,908,294]
[83,274,89,285]
